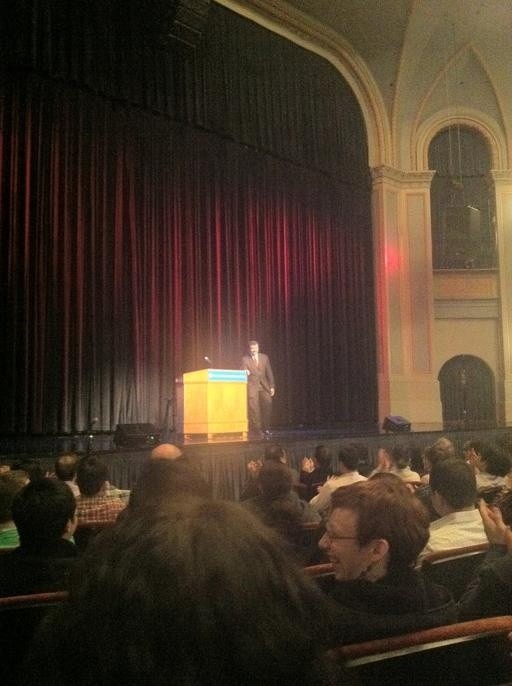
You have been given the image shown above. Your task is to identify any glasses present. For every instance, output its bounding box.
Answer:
[328,533,363,542]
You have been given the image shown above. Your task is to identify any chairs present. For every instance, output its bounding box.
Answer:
[319,616,512,686]
[299,522,321,545]
[0,592,69,675]
[421,543,490,599]
[300,563,336,595]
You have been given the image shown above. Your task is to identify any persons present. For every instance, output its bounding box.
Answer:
[409,458,490,575]
[74,458,128,530]
[240,340,275,436]
[123,455,216,518]
[309,445,370,519]
[239,444,300,501]
[240,458,323,568]
[455,485,512,623]
[310,472,460,652]
[0,477,85,686]
[150,443,182,461]
[300,445,335,502]
[368,442,421,493]
[20,494,361,686]
[350,442,375,478]
[414,446,449,523]
[44,452,81,496]
[0,465,33,548]
[421,436,456,485]
[462,439,483,474]
[469,444,512,492]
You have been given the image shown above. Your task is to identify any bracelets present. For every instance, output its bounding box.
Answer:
[504,525,511,546]
